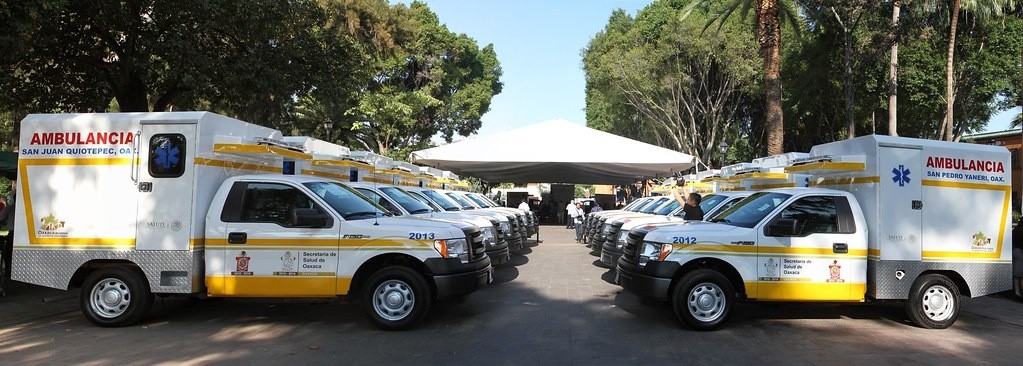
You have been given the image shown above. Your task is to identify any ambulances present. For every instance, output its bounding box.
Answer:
[11,109,495,333]
[584,151,811,296]
[282,135,539,266]
[613,134,1014,333]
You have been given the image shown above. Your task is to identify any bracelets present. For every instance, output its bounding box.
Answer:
[672,186,676,189]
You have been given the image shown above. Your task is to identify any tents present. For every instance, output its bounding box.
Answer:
[410,117,699,185]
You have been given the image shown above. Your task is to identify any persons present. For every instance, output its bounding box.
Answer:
[493,191,501,204]
[518,198,531,211]
[578,201,586,212]
[590,201,603,212]
[1012,216,1023,299]
[670,179,704,220]
[528,200,569,225]
[613,204,623,210]
[570,202,586,243]
[565,200,577,229]
[589,201,595,211]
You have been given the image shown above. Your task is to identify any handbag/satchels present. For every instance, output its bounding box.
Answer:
[577,215,583,221]
